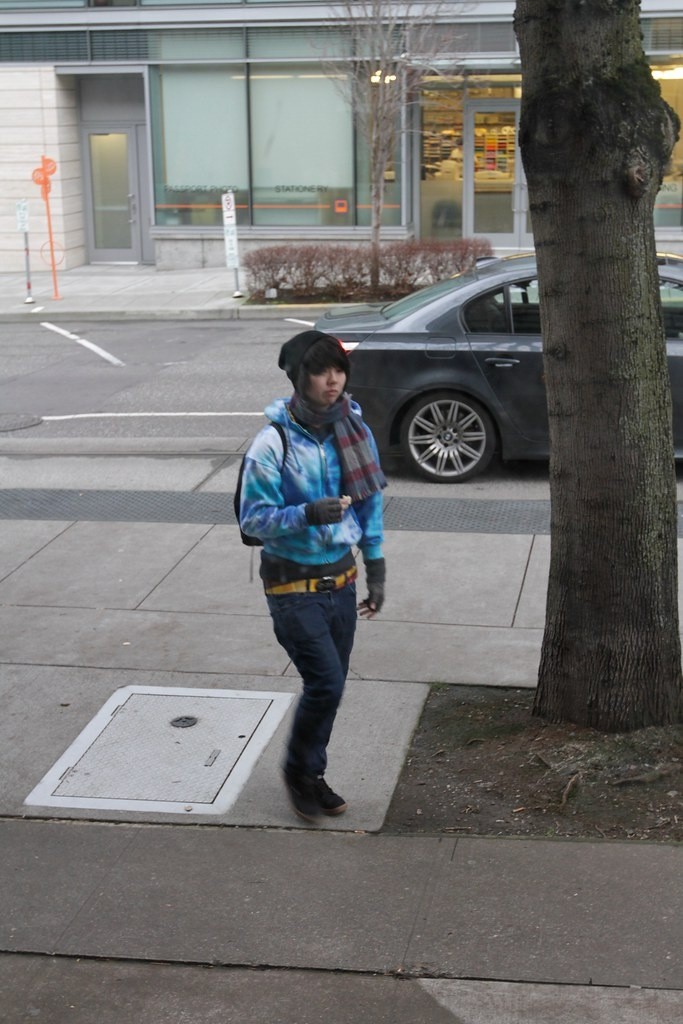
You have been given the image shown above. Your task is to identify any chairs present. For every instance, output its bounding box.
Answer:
[470,296,505,333]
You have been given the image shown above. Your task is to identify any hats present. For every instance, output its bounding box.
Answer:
[279,331,342,396]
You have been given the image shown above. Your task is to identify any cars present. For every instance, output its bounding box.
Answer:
[314,252,683,485]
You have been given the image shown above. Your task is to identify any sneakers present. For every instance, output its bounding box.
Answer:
[284,764,347,824]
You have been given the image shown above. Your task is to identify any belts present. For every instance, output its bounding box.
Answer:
[264,566,358,594]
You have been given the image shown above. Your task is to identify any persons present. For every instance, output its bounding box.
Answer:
[240,330,387,824]
[448,138,478,176]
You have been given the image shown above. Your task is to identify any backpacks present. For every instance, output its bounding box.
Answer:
[234,420,288,546]
